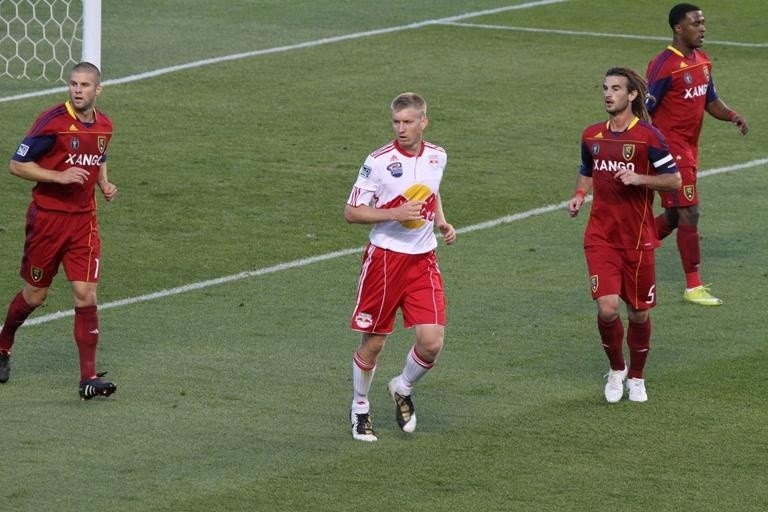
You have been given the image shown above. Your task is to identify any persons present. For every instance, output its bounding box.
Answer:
[345,93,456,442]
[1,62,117,401]
[641,3,748,307]
[569,68,682,403]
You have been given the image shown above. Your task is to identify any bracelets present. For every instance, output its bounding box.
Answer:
[727,111,736,122]
[575,190,585,197]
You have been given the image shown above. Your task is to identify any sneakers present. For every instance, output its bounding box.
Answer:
[684,283,722,306]
[604,363,629,403]
[0,350,11,383]
[387,377,416,433]
[79,371,116,400]
[625,378,648,402]
[349,403,377,441]
